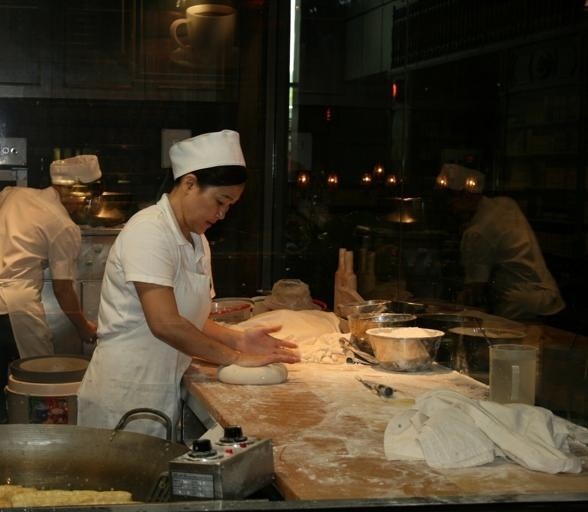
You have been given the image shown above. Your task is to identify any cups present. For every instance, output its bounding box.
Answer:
[169,4,238,51]
[486,343,537,405]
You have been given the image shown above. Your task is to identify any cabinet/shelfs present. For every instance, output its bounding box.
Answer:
[40,279,102,359]
[1,0,218,103]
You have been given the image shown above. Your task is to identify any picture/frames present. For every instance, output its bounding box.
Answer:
[125,1,249,90]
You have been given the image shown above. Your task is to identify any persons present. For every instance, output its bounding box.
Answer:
[433,162,569,321]
[0,154,106,389]
[77,129,302,440]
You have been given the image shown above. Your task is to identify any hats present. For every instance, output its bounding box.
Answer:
[49,154,102,187]
[437,162,488,196]
[169,129,246,182]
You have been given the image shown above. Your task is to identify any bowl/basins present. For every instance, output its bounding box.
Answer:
[335,295,524,369]
[209,296,328,323]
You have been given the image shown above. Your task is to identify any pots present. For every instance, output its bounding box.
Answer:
[0,406,193,507]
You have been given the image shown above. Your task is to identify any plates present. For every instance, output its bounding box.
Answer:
[170,47,225,71]
